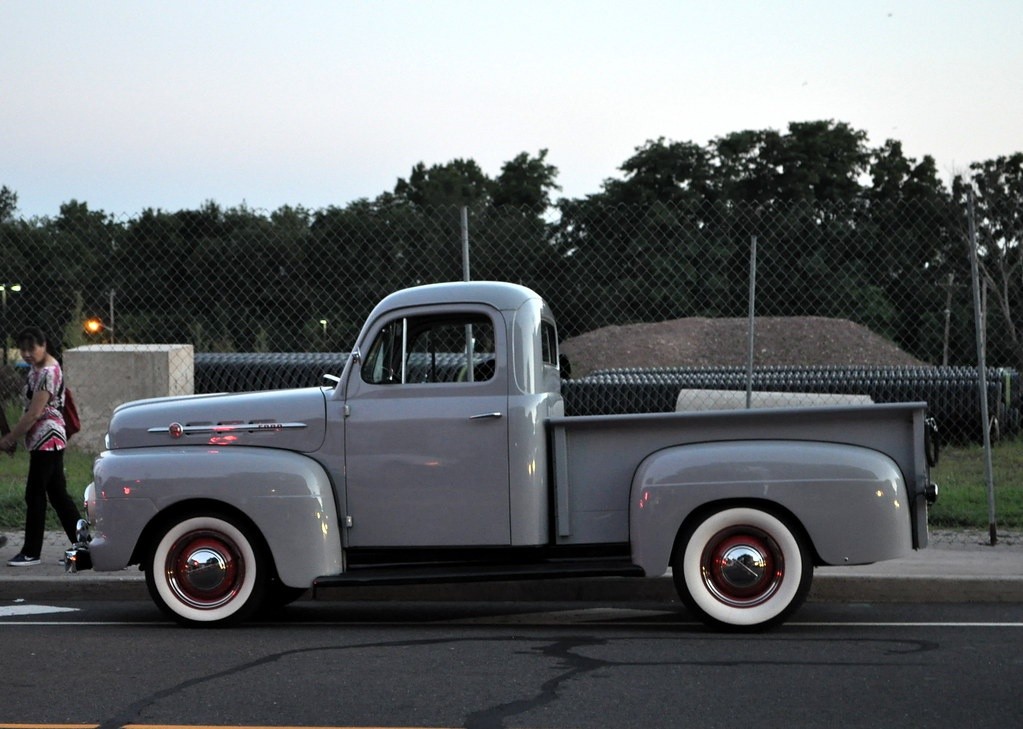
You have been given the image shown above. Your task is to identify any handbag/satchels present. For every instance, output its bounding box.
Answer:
[58,388,81,441]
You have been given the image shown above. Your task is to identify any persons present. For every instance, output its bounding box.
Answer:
[0,326,82,566]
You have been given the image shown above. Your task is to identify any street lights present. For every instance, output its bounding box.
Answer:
[0,283,21,366]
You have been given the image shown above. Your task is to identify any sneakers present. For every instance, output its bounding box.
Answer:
[6,551,41,565]
[59,558,64,565]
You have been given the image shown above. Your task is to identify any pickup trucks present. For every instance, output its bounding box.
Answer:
[62,281,940,629]
